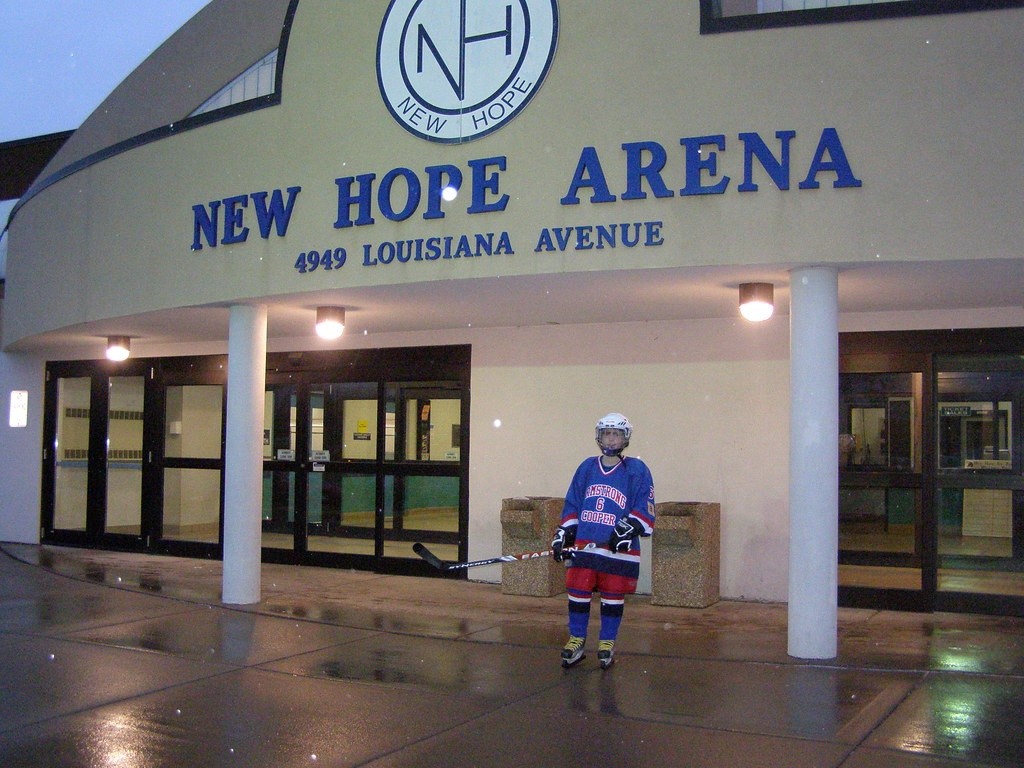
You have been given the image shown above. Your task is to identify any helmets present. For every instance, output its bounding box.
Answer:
[595,413,633,456]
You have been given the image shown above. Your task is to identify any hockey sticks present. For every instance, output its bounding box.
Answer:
[412,541,609,571]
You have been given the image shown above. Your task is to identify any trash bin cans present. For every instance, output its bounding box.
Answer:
[500,496,568,597]
[650,501,721,608]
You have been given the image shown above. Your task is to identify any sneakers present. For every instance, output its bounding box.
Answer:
[561,635,586,668]
[598,640,615,670]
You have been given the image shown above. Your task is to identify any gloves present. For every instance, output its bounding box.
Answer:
[609,514,645,554]
[551,526,576,562]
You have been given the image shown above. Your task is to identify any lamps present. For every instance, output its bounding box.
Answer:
[106,336,130,362]
[739,283,774,322]
[316,307,345,341]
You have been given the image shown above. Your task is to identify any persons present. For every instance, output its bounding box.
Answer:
[551,412,655,660]
